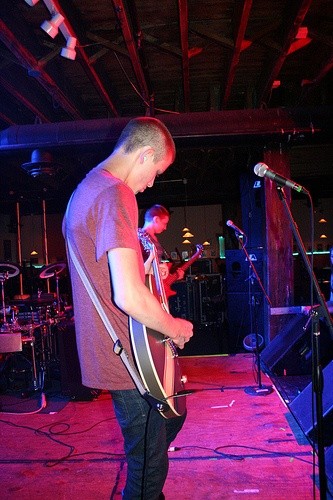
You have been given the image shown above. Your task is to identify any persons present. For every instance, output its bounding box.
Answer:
[139,205,185,296]
[62,118,194,500]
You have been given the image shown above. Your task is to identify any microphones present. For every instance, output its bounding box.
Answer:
[226,219,245,236]
[253,163,304,193]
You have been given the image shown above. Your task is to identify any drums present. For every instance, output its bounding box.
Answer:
[0,299,60,325]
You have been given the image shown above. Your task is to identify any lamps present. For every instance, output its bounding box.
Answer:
[24,0,40,6]
[181,226,210,246]
[41,12,64,39]
[61,37,77,60]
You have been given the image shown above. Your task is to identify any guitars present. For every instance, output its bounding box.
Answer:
[129,228,187,419]
[149,244,204,304]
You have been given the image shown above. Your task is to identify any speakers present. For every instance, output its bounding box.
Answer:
[288,359,333,447]
[58,322,101,401]
[256,312,333,378]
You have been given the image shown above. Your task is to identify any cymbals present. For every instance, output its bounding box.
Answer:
[39,261,67,279]
[0,263,21,280]
